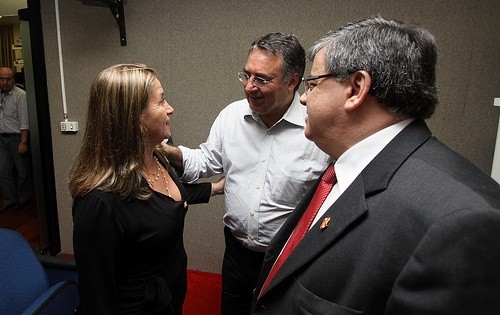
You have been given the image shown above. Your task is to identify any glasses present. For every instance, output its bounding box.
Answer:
[236,72,282,89]
[301,69,365,96]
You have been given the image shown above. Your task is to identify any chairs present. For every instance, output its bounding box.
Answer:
[0,227,82,315]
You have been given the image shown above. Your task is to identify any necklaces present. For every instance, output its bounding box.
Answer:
[142,156,172,197]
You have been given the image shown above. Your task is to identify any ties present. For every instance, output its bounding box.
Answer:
[256,162,337,299]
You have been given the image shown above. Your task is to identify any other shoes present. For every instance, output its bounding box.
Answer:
[14,201,31,209]
[0,201,15,213]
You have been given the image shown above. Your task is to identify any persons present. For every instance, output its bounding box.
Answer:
[155,32,336,315]
[68,64,226,315]
[243,13,500,315]
[0,66,30,230]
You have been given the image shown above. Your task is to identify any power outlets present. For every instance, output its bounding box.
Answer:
[60,121,78,132]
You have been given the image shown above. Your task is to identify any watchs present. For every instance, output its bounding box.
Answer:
[20,140,27,144]
